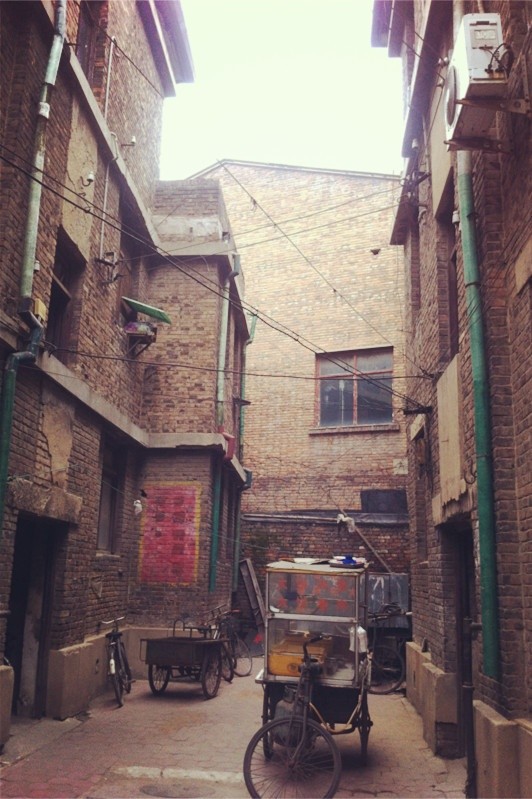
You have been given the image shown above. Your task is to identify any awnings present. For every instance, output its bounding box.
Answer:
[121,296,170,326]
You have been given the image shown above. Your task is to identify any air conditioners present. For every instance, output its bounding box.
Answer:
[443,12,503,143]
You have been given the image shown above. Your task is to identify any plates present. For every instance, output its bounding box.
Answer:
[294,557,317,564]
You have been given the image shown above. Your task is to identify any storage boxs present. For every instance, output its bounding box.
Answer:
[269,632,332,678]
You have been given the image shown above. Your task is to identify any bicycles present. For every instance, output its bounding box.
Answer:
[97,610,143,706]
[227,618,252,676]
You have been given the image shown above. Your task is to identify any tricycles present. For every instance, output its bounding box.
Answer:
[368,573,411,696]
[243,557,373,799]
[140,603,234,699]
